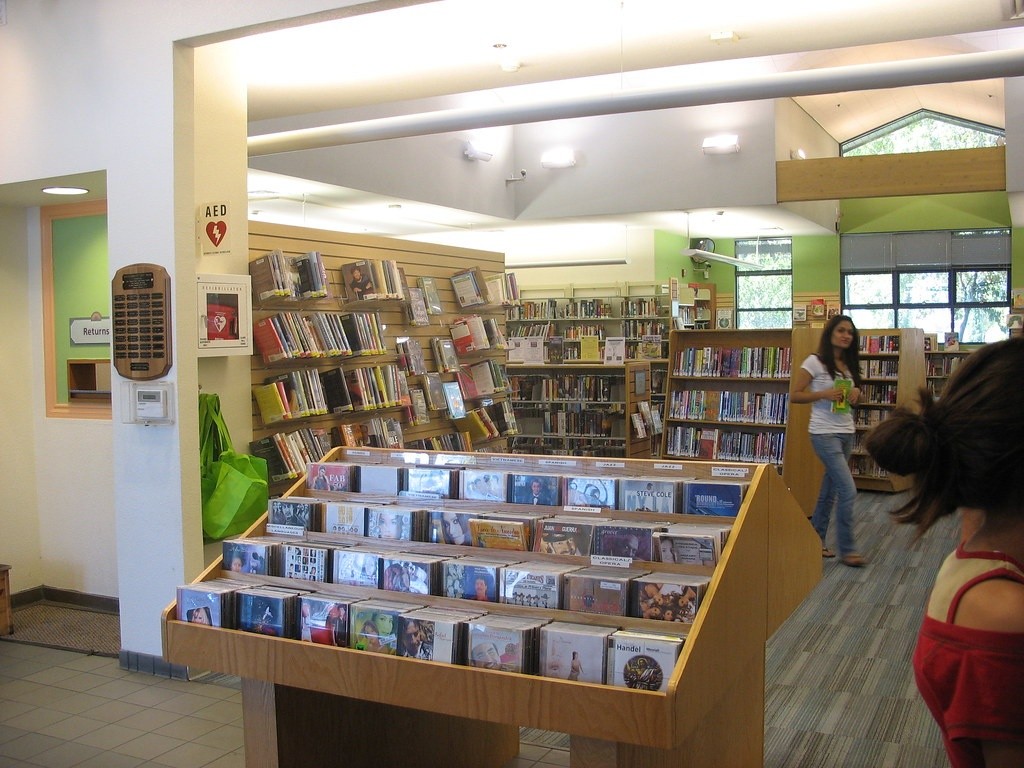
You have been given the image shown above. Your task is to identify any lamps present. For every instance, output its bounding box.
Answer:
[680,249,763,269]
[790,148,805,160]
[464,138,492,162]
[702,133,740,156]
[505,258,631,269]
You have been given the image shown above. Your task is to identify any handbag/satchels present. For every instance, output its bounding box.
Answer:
[199,393,270,546]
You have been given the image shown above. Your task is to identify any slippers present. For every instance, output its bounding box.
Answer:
[838,551,868,567]
[822,547,836,558]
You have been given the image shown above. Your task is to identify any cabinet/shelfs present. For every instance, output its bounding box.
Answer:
[662,328,927,495]
[247,221,522,500]
[162,447,823,768]
[660,283,716,329]
[505,277,680,458]
[923,348,973,401]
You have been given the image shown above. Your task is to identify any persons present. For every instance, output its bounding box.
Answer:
[568,651,584,681]
[348,266,373,300]
[313,465,330,489]
[671,586,696,609]
[436,513,471,547]
[371,611,396,654]
[662,609,675,621]
[223,542,248,572]
[361,620,380,652]
[279,502,303,526]
[609,534,640,559]
[332,604,348,646]
[639,581,666,607]
[641,601,664,620]
[543,522,585,555]
[868,337,1024,768]
[469,578,490,601]
[397,618,433,661]
[470,628,502,670]
[790,314,866,567]
[377,513,403,539]
[524,479,550,505]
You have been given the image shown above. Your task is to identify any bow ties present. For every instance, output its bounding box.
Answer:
[534,496,538,499]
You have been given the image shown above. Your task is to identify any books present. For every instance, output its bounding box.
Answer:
[178,249,790,692]
[849,331,968,478]
[831,378,853,415]
